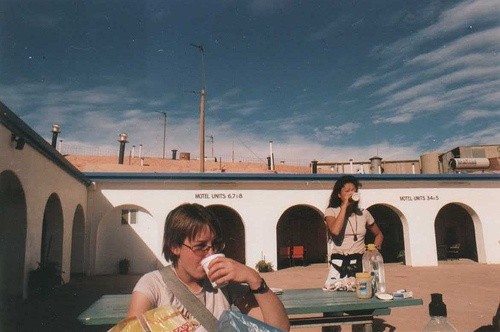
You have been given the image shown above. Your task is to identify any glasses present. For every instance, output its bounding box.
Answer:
[181,242,225,257]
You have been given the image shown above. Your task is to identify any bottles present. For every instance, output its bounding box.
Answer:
[421,293,458,332]
[362,244,386,294]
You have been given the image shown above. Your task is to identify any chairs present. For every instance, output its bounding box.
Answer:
[279,246,305,267]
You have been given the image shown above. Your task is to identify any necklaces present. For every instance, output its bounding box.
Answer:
[340,204,358,241]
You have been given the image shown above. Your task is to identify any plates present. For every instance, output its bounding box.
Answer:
[269,288,283,295]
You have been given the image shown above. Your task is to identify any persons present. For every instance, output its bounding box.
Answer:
[321,175,384,332]
[127,203,290,329]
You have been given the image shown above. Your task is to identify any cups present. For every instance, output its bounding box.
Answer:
[351,192,360,201]
[200,253,229,289]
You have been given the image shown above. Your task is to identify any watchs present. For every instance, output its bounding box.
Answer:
[250,279,269,294]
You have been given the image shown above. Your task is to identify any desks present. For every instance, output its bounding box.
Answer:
[76,288,424,332]
